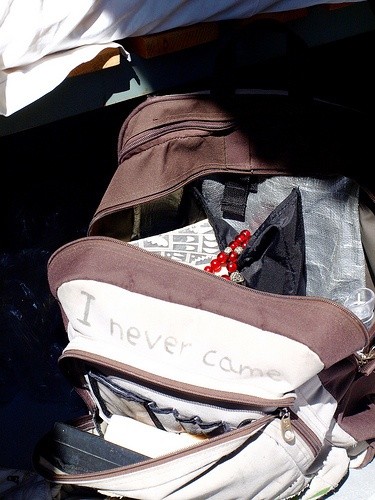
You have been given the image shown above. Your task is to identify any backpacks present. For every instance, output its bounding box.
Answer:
[9,78,375,500]
